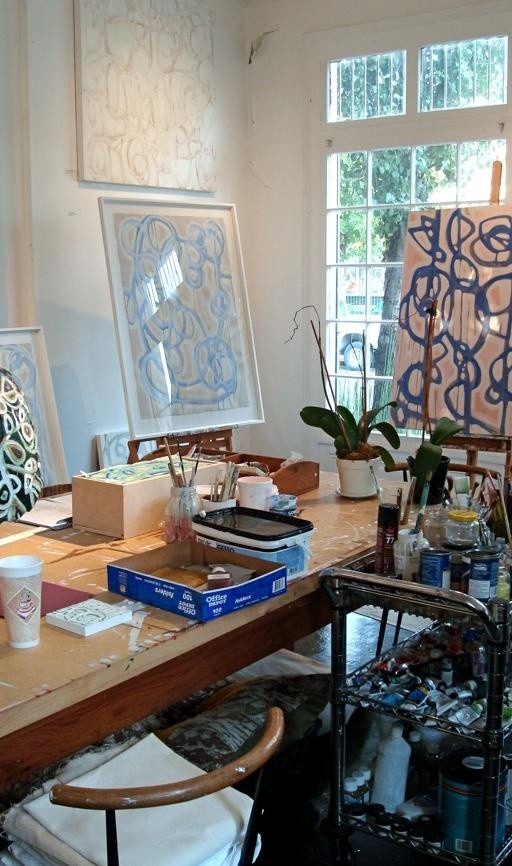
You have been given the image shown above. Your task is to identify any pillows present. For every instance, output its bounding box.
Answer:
[153,671,336,790]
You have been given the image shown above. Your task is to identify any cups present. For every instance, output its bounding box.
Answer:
[1,555,43,649]
[269,506,297,518]
[201,493,237,513]
[268,494,298,511]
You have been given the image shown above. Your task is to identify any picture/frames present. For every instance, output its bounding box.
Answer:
[0,325,70,494]
[95,192,269,430]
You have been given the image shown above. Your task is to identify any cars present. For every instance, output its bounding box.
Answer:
[338,299,385,371]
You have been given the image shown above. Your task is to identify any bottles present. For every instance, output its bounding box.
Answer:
[437,658,454,687]
[343,721,449,821]
[393,505,512,602]
[375,503,400,576]
[164,485,202,544]
[464,628,489,680]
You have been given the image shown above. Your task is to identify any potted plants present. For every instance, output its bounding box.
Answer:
[405,410,468,505]
[285,304,402,500]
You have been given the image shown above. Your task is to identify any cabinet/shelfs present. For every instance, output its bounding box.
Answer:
[317,530,512,864]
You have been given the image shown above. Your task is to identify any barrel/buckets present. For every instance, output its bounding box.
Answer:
[407,454,450,504]
[337,455,385,498]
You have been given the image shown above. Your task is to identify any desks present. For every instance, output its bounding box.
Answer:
[2,446,454,797]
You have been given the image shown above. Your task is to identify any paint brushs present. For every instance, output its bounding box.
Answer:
[466,468,511,548]
[163,433,204,489]
[210,460,240,501]
[396,476,420,526]
[371,464,385,506]
[415,467,433,533]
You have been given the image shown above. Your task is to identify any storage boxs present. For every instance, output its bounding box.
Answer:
[189,505,314,582]
[68,453,232,541]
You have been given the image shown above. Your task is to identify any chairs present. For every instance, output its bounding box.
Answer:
[376,456,503,660]
[3,705,289,866]
[0,367,238,788]
